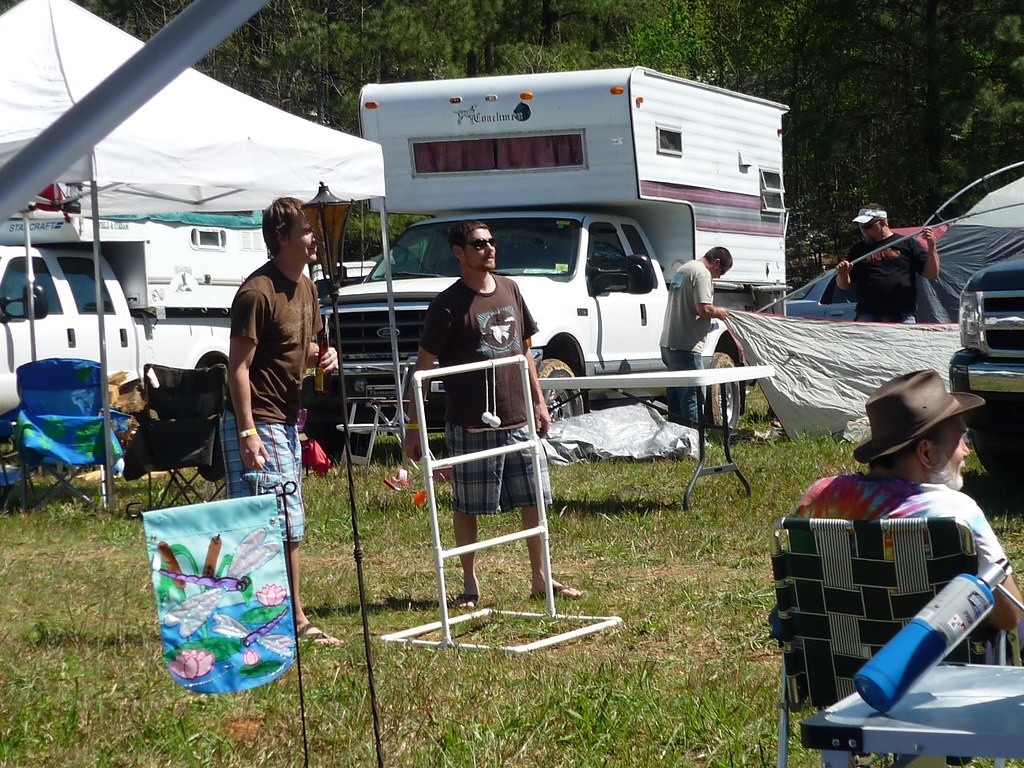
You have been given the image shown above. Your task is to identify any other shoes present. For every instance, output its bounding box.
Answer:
[820,748,854,767]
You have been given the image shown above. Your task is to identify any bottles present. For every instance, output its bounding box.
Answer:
[315,333,331,396]
[853,559,1013,714]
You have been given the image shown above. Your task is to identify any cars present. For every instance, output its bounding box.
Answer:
[787,268,857,323]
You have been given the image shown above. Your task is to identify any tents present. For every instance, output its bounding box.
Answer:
[0,0,405,514]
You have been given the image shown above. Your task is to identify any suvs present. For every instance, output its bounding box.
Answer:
[947,254,1024,478]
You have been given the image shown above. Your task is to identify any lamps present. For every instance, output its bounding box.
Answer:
[300,182,354,293]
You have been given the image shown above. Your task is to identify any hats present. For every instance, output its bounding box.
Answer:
[852,209,888,224]
[853,369,985,465]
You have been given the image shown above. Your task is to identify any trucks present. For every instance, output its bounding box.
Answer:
[304,66,794,441]
[0,202,374,488]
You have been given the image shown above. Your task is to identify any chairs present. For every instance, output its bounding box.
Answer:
[766,516,1024,768]
[511,236,545,268]
[0,358,134,516]
[124,362,229,523]
[335,356,436,471]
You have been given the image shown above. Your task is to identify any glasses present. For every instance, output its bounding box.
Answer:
[468,238,496,250]
[858,220,878,230]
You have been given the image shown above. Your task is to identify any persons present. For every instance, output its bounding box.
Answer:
[658,247,733,427]
[405,220,582,605]
[218,197,344,647]
[836,203,940,323]
[796,369,1024,629]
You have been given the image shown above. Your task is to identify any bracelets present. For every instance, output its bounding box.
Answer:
[239,428,257,438]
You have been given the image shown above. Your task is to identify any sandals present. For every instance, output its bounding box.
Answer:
[296,623,344,649]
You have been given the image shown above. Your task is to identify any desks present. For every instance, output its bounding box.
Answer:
[432,364,776,512]
[800,662,1024,768]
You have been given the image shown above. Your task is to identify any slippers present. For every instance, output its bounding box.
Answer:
[452,593,480,610]
[531,587,585,601]
